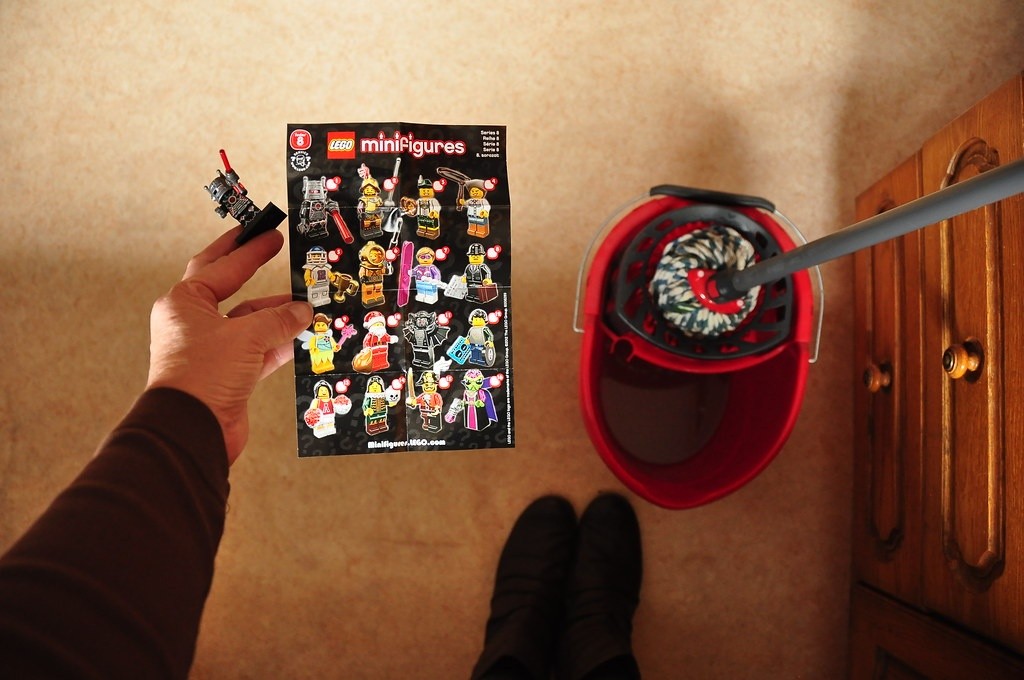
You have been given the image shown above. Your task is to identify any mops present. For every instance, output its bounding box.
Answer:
[645,158,1024,341]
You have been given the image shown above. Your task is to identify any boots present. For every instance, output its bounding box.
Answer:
[561,492,643,680]
[470,495,576,680]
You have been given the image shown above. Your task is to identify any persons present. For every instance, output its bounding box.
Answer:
[0,224,643,680]
